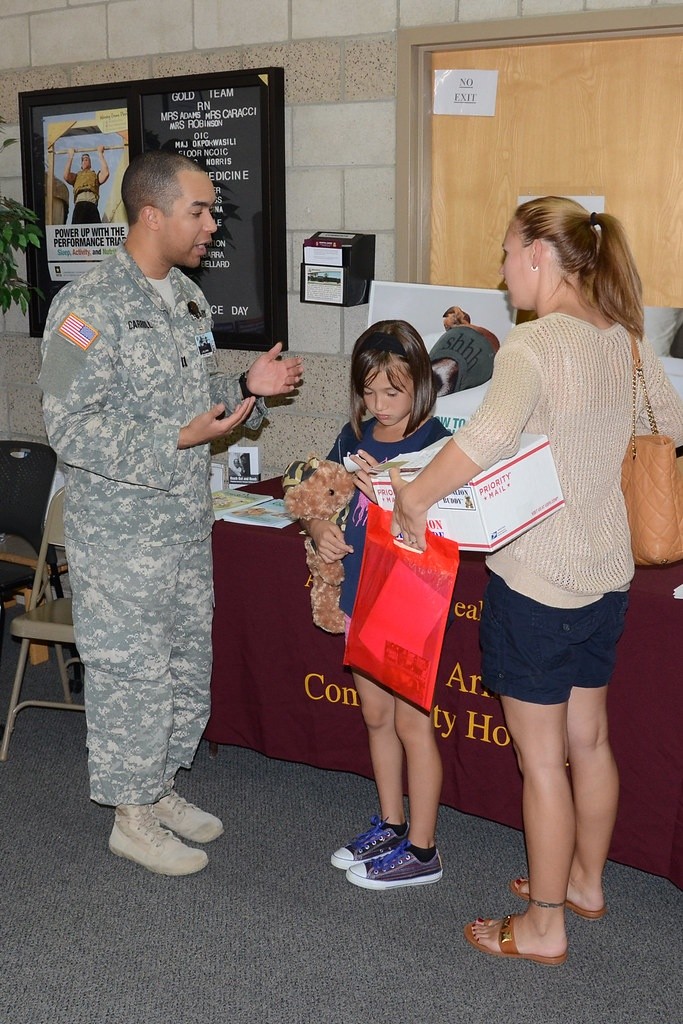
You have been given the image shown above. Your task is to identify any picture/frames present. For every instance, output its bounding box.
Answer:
[22,67,287,351]
[367,281,517,399]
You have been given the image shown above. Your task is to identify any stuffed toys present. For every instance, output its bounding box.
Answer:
[284,455,351,635]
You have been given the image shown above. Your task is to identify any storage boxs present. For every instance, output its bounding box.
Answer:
[372,434,566,552]
[300,231,375,307]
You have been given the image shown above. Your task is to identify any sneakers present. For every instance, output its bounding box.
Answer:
[108,805,209,877]
[330,815,411,871]
[153,789,225,843]
[345,840,443,890]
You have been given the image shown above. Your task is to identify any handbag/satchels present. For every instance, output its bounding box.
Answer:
[340,499,459,716]
[620,434,683,568]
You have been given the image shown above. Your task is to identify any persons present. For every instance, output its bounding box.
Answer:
[65,145,110,225]
[300,320,443,888]
[38,151,303,875]
[391,197,683,964]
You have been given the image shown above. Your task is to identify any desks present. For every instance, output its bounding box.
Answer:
[202,469,682,887]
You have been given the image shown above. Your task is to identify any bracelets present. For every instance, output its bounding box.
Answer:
[237,372,260,400]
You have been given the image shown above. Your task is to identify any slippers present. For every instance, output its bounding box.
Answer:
[463,914,569,966]
[509,874,608,920]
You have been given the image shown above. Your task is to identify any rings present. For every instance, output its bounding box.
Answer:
[408,539,417,545]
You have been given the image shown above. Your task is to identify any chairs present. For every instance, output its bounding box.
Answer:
[0,441,65,660]
[1,486,86,761]
